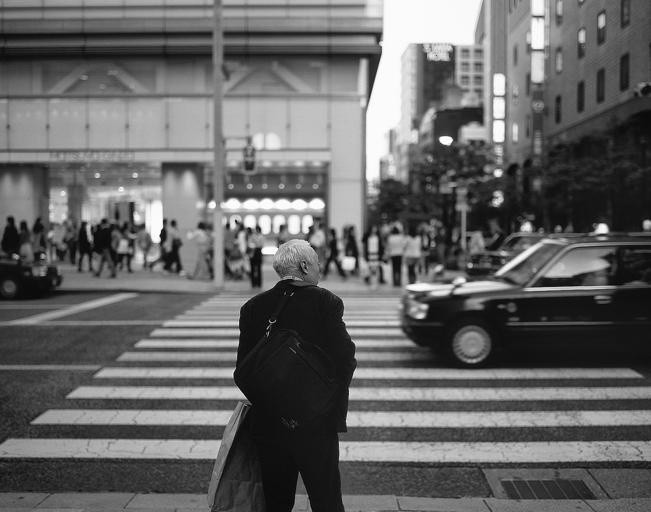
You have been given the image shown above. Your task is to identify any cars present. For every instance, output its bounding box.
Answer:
[461,229,572,288]
[0,249,62,298]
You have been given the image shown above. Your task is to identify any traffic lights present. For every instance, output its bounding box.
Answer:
[634,81,651,100]
[242,144,258,176]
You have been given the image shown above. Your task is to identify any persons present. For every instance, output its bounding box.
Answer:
[234,238,356,510]
[1,214,267,288]
[311,220,613,288]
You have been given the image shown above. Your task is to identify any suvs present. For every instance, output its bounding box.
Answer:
[397,231,651,368]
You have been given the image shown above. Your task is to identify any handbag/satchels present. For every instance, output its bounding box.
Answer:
[234,329,339,432]
